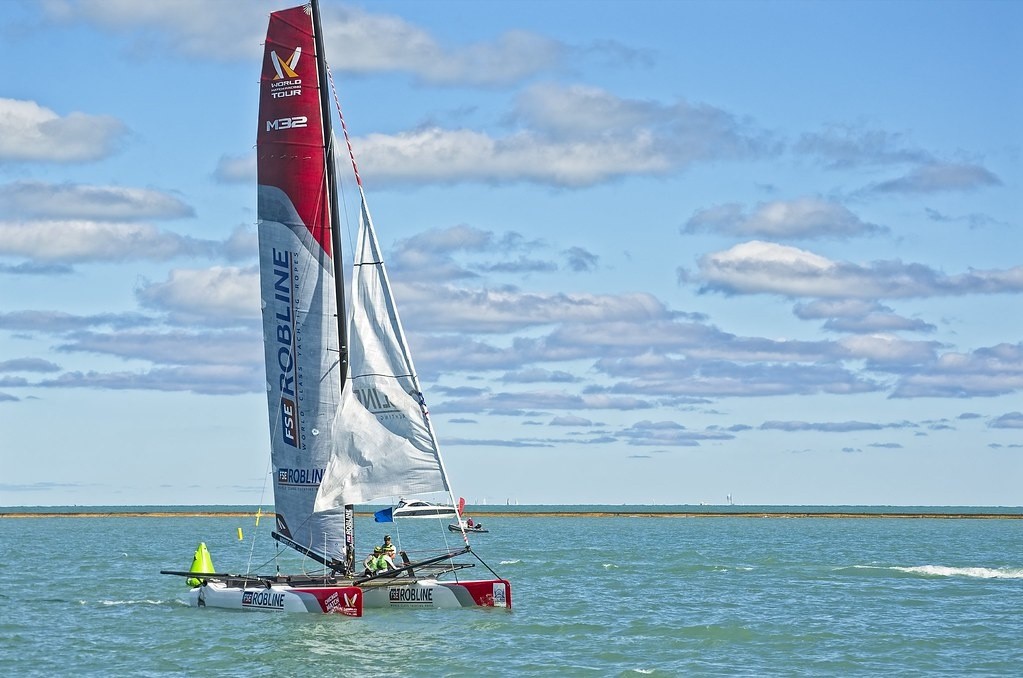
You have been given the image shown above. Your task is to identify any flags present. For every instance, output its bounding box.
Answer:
[374,507,393,522]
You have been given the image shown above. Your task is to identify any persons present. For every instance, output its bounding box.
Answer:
[363,535,400,577]
[467,517,473,527]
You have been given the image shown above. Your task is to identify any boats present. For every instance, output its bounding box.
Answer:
[390,497,459,521]
[448,524,490,534]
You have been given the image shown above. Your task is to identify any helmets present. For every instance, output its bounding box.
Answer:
[374,546,382,553]
[385,535,391,539]
[384,546,393,551]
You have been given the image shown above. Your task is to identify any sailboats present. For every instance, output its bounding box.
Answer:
[159,0,512,618]
